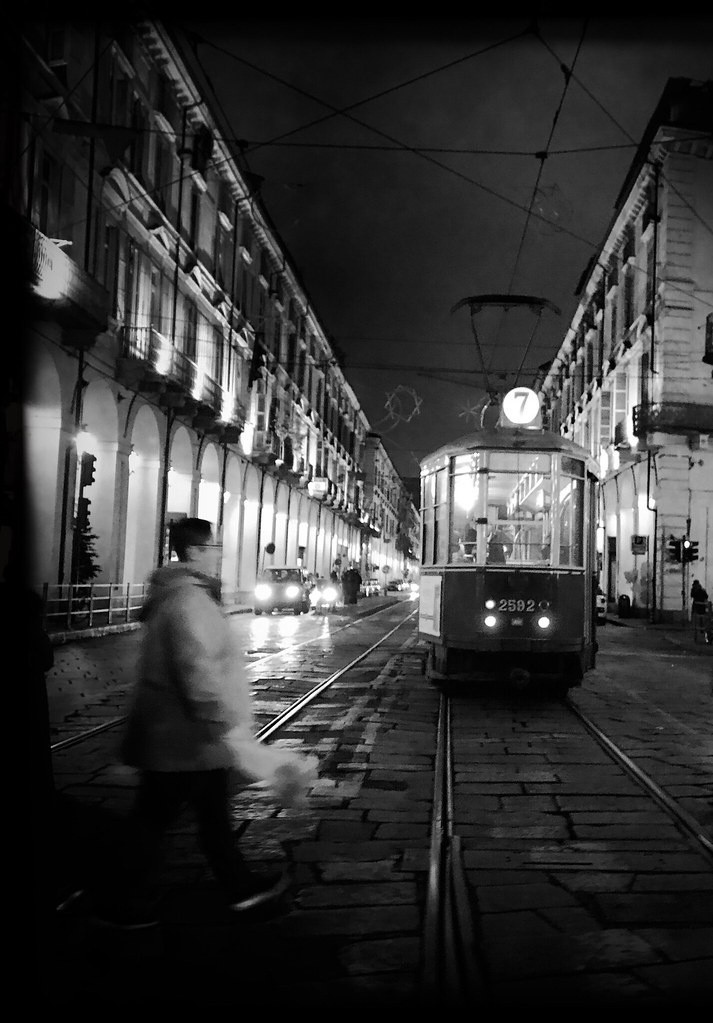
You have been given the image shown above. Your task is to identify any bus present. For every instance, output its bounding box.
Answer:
[421,426,601,702]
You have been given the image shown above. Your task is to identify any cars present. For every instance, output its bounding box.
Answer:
[254,567,312,616]
[596,587,608,626]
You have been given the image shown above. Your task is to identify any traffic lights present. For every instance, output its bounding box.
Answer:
[683,540,692,563]
[689,541,699,562]
[670,539,683,563]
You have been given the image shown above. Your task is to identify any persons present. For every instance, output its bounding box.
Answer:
[453,515,568,566]
[326,565,344,613]
[115,517,309,914]
[689,579,708,632]
[206,571,363,606]
